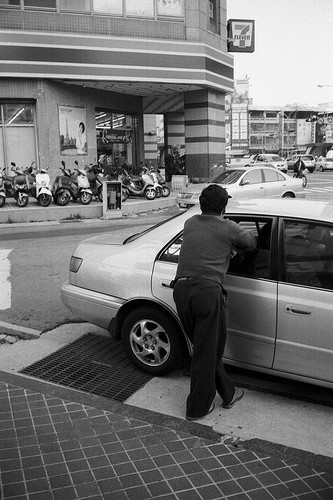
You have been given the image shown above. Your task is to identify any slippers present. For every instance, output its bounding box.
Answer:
[186,403,215,420]
[222,388,244,409]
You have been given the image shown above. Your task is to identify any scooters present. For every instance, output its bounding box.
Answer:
[0,154,171,207]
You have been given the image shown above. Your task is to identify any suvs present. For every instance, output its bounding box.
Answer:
[285,154,316,172]
[247,154,288,174]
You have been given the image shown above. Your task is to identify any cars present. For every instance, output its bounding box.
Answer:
[177,166,307,209]
[59,197,333,377]
[315,158,333,172]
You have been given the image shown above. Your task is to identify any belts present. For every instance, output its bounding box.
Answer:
[178,277,192,280]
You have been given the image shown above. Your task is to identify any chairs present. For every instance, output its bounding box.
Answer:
[305,228,333,280]
[284,234,322,289]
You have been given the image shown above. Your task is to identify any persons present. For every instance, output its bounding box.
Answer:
[165,147,180,182]
[76,122,87,154]
[295,157,305,178]
[173,185,256,419]
